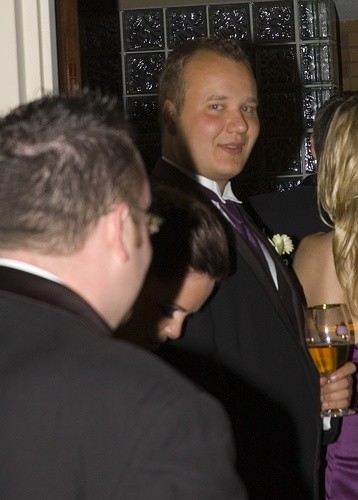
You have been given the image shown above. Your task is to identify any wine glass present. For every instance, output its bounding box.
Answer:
[305,303,356,417]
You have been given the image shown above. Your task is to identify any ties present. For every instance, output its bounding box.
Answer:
[198,184,278,294]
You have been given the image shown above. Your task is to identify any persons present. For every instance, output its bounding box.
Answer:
[292,98,358,500]
[242,90,358,265]
[0,88,233,500]
[127,37,356,500]
[115,184,229,353]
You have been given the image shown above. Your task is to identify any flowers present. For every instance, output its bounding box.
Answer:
[268,234,294,255]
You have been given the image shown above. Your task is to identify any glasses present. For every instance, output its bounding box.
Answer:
[103,203,163,235]
